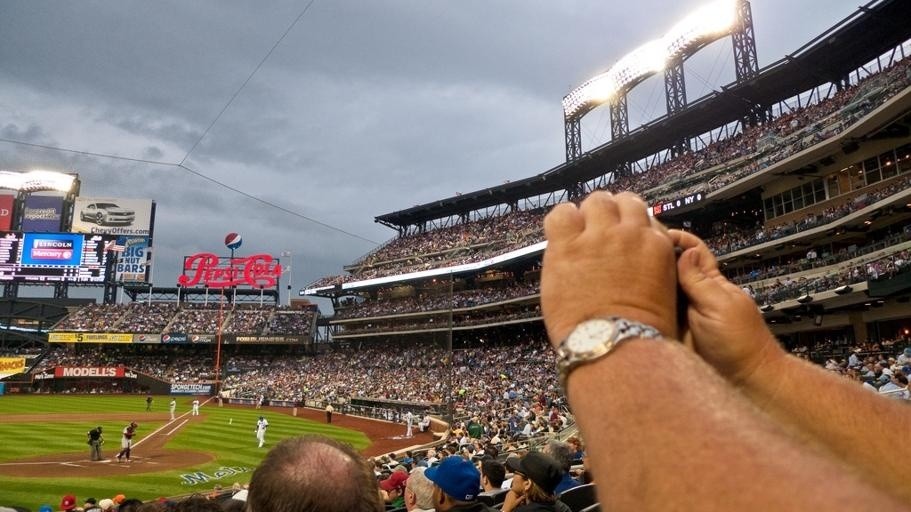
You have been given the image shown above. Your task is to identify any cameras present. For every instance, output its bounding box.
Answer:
[674,242,689,338]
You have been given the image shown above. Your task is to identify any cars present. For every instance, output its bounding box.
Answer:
[81,202,136,226]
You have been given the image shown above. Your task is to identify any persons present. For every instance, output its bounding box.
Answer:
[293,399,298,416]
[1,302,301,405]
[218,392,224,408]
[145,396,154,413]
[59,438,380,510]
[540,191,911,507]
[115,420,138,463]
[254,416,269,448]
[301,59,911,511]
[192,396,199,416]
[256,392,264,409]
[325,402,334,424]
[87,426,106,461]
[418,413,431,432]
[169,397,176,420]
[404,410,413,436]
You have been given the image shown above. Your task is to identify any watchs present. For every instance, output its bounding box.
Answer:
[554,317,664,411]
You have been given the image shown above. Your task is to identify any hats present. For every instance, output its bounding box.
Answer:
[60,494,125,510]
[507,451,563,485]
[424,456,480,500]
[380,471,409,490]
